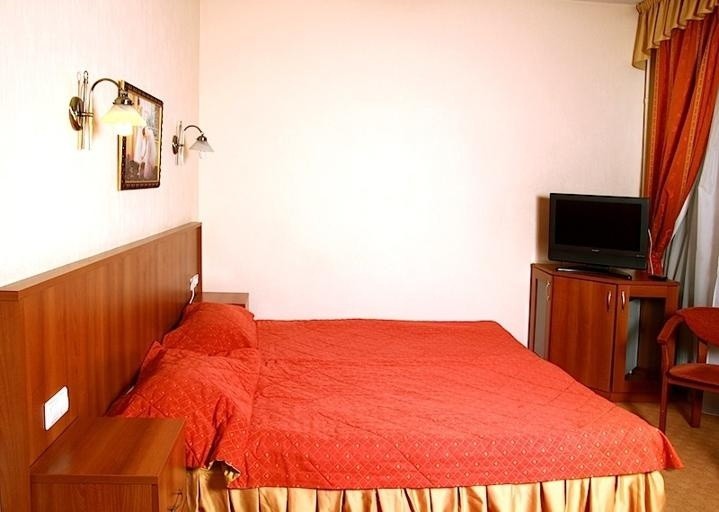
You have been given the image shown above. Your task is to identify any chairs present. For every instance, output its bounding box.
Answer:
[656,306,719,435]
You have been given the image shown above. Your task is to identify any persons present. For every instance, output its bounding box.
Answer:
[131,106,148,177]
[140,127,158,178]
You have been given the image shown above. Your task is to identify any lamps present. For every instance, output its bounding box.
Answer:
[69,70,148,151]
[170,119,215,166]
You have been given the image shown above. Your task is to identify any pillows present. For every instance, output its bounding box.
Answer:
[162,303,259,355]
[105,341,261,471]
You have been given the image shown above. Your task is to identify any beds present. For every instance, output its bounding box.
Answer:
[0,221,687,512]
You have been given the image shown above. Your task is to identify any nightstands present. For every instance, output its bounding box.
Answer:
[191,291,249,311]
[30,415,188,512]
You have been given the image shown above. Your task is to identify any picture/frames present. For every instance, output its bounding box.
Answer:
[117,80,164,191]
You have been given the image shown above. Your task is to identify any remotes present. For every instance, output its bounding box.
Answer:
[649,274,668,281]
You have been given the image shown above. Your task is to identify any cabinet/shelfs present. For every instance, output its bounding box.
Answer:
[528,262,679,403]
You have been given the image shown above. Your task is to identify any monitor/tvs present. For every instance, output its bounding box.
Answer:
[548,193,650,280]
